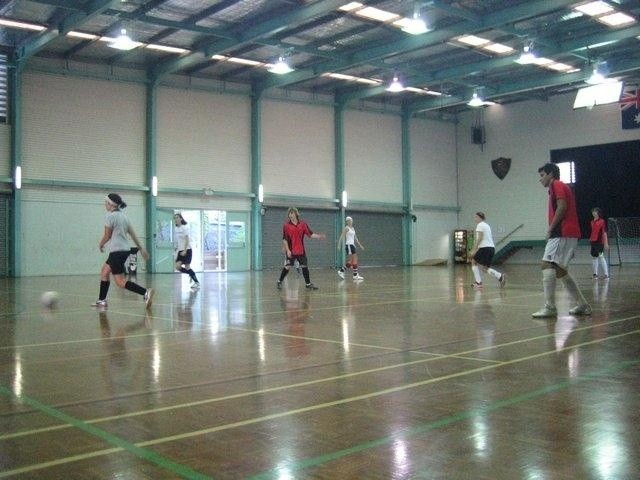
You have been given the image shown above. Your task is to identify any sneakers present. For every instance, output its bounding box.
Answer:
[337,270,344,279]
[568,303,593,314]
[305,282,318,291]
[471,281,484,288]
[531,304,561,319]
[498,272,507,288]
[591,272,600,280]
[276,279,283,290]
[189,273,200,289]
[143,286,154,308]
[353,272,363,280]
[89,299,110,310]
[598,273,610,280]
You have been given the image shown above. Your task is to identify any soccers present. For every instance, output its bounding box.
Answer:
[42,292,58,306]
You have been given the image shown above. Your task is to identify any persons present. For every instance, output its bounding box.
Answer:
[337,216,366,281]
[92,193,154,309]
[531,163,592,317]
[536,318,589,385]
[276,289,312,363]
[474,289,496,362]
[276,207,326,291]
[174,214,199,289]
[589,207,609,279]
[468,212,506,288]
[590,279,610,319]
[177,289,198,332]
[94,309,154,392]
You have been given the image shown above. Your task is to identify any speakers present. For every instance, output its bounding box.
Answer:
[471,127,485,143]
